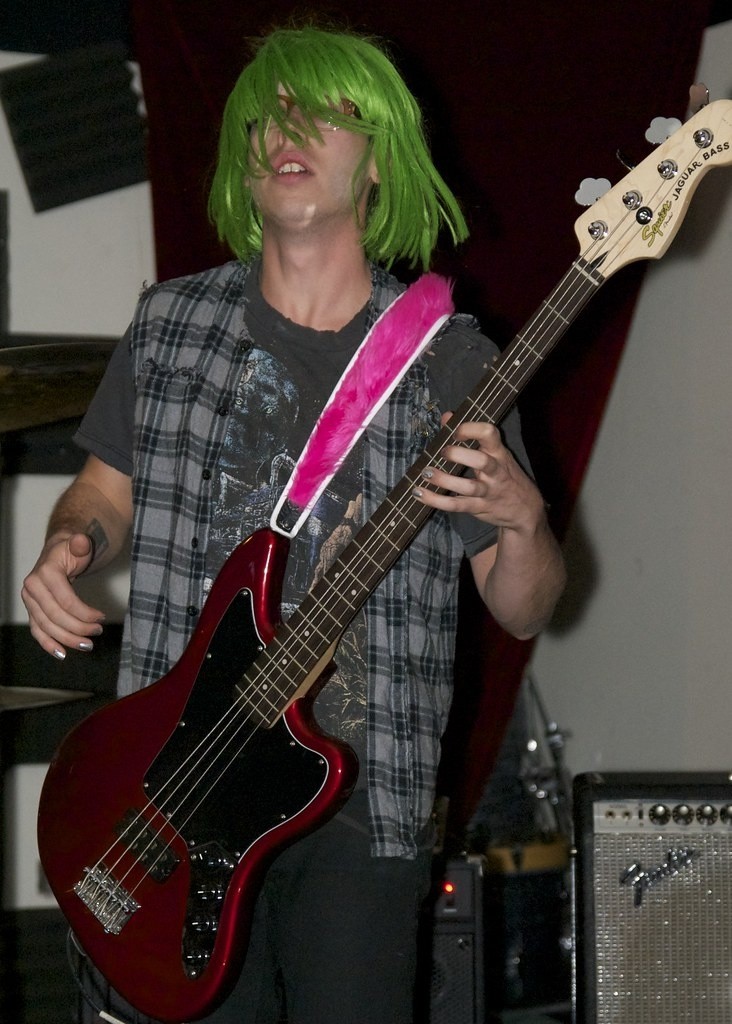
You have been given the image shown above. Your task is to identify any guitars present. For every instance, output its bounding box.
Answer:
[37,95,732,1024]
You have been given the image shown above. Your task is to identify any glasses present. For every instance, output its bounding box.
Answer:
[253,94,356,131]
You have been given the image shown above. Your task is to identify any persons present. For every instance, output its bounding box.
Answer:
[16,11,570,1024]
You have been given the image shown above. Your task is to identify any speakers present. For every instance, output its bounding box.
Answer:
[572,770,732,1024]
[413,852,489,1024]
